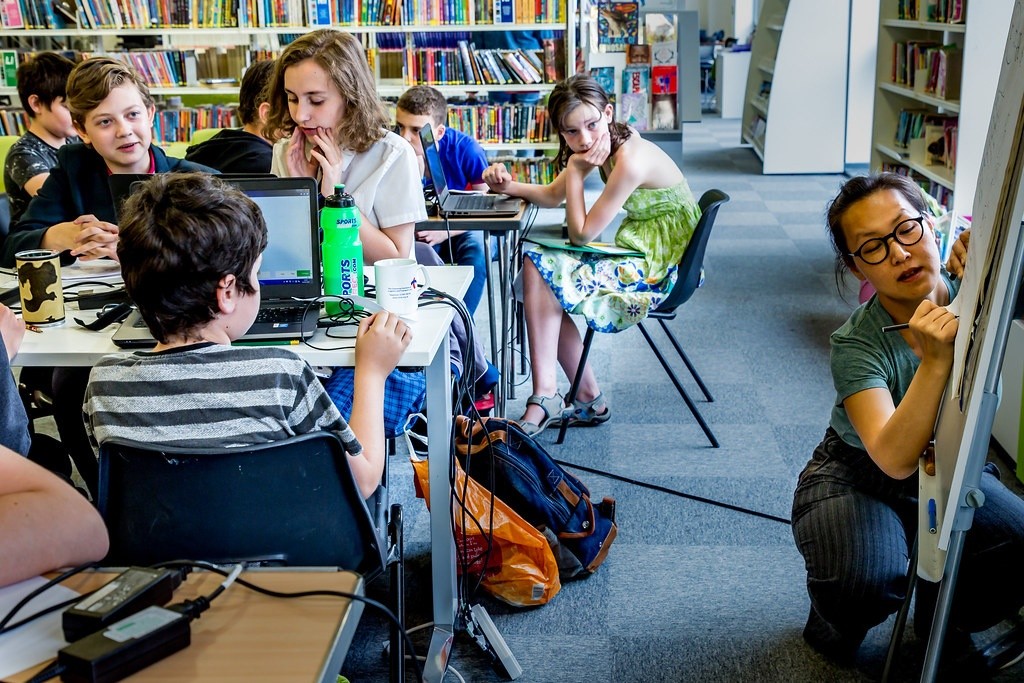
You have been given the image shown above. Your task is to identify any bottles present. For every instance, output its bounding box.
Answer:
[319,183,365,316]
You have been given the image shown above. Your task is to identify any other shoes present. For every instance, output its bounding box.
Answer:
[918,628,977,662]
[802,614,859,667]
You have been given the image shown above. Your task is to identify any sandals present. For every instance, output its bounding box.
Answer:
[550,392,611,426]
[516,394,574,439]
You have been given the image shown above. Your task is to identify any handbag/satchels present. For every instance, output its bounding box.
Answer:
[404,414,562,607]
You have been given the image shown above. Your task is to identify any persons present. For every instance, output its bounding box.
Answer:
[397,88,490,317]
[481,74,704,438]
[792,173,971,663]
[0,29,429,588]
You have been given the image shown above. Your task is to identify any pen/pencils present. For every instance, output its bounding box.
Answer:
[25,324,43,333]
[882,316,960,333]
[231,340,299,346]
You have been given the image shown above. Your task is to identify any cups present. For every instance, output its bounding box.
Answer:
[372,257,431,319]
[14,248,67,328]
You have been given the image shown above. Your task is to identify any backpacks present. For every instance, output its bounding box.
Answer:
[454,415,616,577]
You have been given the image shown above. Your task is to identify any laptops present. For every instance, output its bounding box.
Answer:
[108,173,321,347]
[417,123,520,215]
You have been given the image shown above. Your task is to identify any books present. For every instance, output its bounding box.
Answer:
[757,80,772,102]
[747,114,765,153]
[881,162,954,261]
[485,155,558,186]
[897,1,966,26]
[892,40,962,101]
[1,34,567,83]
[0,96,560,144]
[894,108,957,173]
[1,0,569,29]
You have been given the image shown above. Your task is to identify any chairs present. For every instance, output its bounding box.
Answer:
[556,189,731,447]
[98,431,404,683]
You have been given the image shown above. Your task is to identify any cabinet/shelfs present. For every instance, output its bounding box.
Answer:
[741,0,852,175]
[0,0,588,238]
[869,0,1017,262]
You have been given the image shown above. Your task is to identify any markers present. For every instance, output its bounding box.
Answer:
[928,499,937,534]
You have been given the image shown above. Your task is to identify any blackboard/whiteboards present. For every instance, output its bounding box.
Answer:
[915,0,1024,582]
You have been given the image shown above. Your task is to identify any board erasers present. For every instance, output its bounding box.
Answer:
[925,443,935,475]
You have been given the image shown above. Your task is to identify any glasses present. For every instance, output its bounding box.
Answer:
[847,213,924,265]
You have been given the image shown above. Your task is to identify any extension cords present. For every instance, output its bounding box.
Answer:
[472,604,523,681]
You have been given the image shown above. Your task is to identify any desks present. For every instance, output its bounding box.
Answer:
[0,570,364,683]
[0,265,476,683]
[415,192,533,416]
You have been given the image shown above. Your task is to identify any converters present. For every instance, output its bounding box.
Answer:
[77,287,134,309]
[62,566,172,642]
[57,603,191,683]
